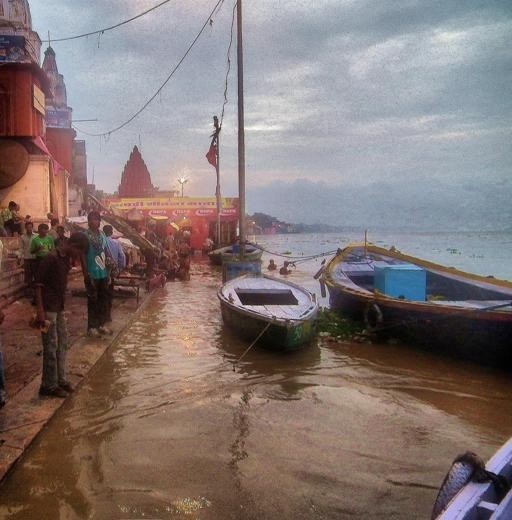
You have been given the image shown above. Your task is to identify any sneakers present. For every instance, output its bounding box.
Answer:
[38,381,75,399]
[87,327,113,341]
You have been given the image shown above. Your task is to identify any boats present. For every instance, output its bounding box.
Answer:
[431,437,512,519]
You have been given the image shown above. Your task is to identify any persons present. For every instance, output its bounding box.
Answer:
[30,231,90,398]
[267,259,278,271]
[79,209,114,338]
[0,200,128,323]
[279,260,289,275]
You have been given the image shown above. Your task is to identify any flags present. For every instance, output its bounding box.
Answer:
[204,132,220,167]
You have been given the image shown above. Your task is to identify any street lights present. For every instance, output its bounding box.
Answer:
[177,178,188,198]
